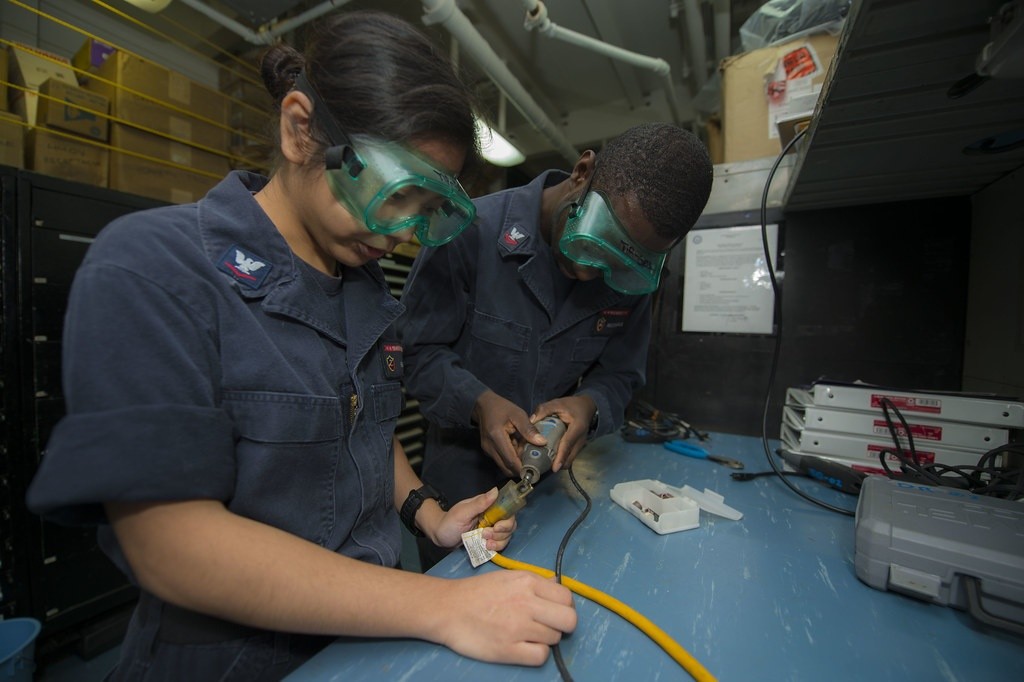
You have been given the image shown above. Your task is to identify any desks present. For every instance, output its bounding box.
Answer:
[283,424,1024,682]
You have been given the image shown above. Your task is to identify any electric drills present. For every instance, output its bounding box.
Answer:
[519,414,567,491]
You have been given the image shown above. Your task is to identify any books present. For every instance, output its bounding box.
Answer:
[781,385,1024,484]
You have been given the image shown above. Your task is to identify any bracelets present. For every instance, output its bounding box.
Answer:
[400,486,450,538]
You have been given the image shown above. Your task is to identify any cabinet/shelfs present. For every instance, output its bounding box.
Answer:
[0,165,179,682]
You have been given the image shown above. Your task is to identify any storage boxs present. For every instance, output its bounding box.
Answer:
[854,475,1024,634]
[709,33,839,166]
[611,478,743,535]
[1,37,278,206]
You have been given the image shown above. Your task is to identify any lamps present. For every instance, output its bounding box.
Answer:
[472,111,526,168]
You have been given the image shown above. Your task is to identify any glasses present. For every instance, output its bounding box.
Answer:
[558,191,670,295]
[326,140,477,248]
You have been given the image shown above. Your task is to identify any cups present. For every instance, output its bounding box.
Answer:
[0,617,41,682]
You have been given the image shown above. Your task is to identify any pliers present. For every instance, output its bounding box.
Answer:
[664,439,744,469]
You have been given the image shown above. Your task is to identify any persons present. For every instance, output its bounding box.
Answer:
[27,11,575,682]
[401,121,714,487]
